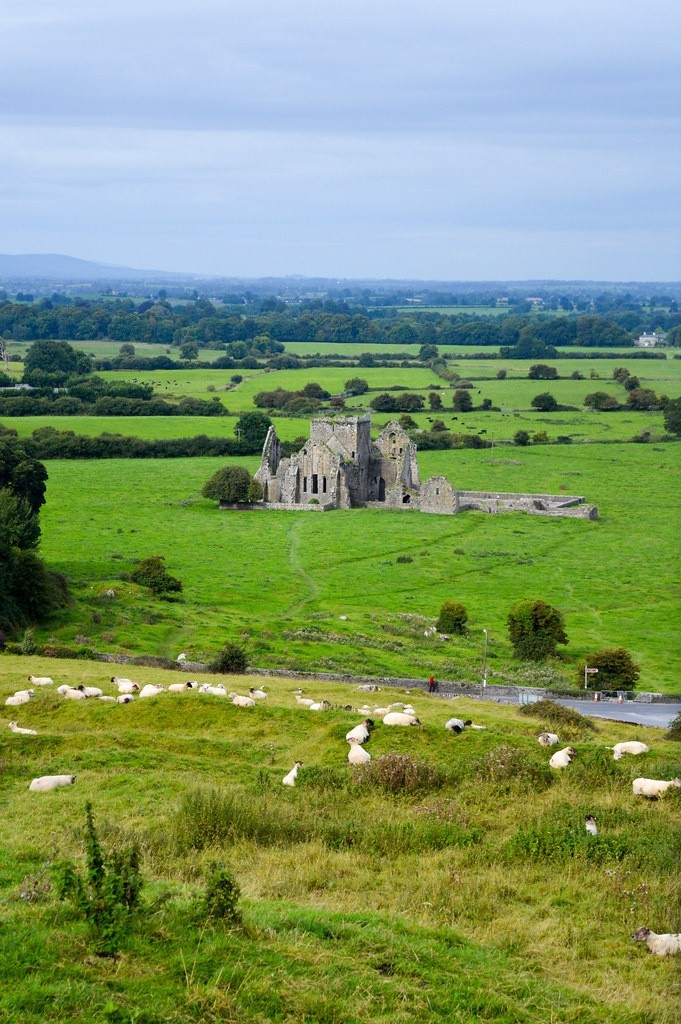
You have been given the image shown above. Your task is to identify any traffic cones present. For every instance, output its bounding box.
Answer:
[593,692,598,702]
[618,694,622,703]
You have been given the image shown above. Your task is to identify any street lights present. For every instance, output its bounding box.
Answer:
[481,629,488,696]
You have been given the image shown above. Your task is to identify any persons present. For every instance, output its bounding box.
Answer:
[429,674,436,694]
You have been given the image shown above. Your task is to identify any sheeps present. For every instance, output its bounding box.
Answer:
[282,760,303,787]
[168,681,198,692]
[111,677,164,698]
[347,738,370,765]
[56,684,134,704]
[198,683,255,708]
[28,675,53,686]
[248,687,267,700]
[342,702,422,745]
[631,927,681,956]
[29,775,75,792]
[8,720,37,734]
[586,815,597,835]
[612,741,648,759]
[632,777,681,798]
[294,695,314,705]
[5,689,34,705]
[538,732,577,769]
[310,700,330,711]
[444,718,472,733]
